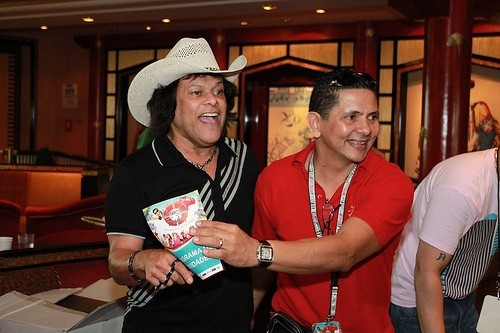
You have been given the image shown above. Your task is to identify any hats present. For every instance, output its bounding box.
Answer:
[127,38,248,128]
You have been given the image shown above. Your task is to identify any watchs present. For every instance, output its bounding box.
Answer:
[257,239,274,271]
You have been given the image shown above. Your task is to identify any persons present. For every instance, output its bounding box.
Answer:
[189,69,415,333]
[390,145,500,333]
[103,37,262,333]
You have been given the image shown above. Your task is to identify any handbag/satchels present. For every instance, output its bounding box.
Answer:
[265,312,306,333]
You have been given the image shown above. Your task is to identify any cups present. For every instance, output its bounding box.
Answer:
[17,233,34,248]
[0,237,13,251]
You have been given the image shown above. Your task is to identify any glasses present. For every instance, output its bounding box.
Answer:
[322,200,341,237]
[315,71,373,111]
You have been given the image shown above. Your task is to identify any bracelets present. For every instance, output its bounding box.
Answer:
[128,249,147,283]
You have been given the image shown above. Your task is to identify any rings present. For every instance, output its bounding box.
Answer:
[154,282,162,290]
[167,267,175,278]
[218,238,223,249]
[162,278,170,286]
[172,259,180,267]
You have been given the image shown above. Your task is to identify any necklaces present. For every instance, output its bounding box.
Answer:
[187,145,217,169]
